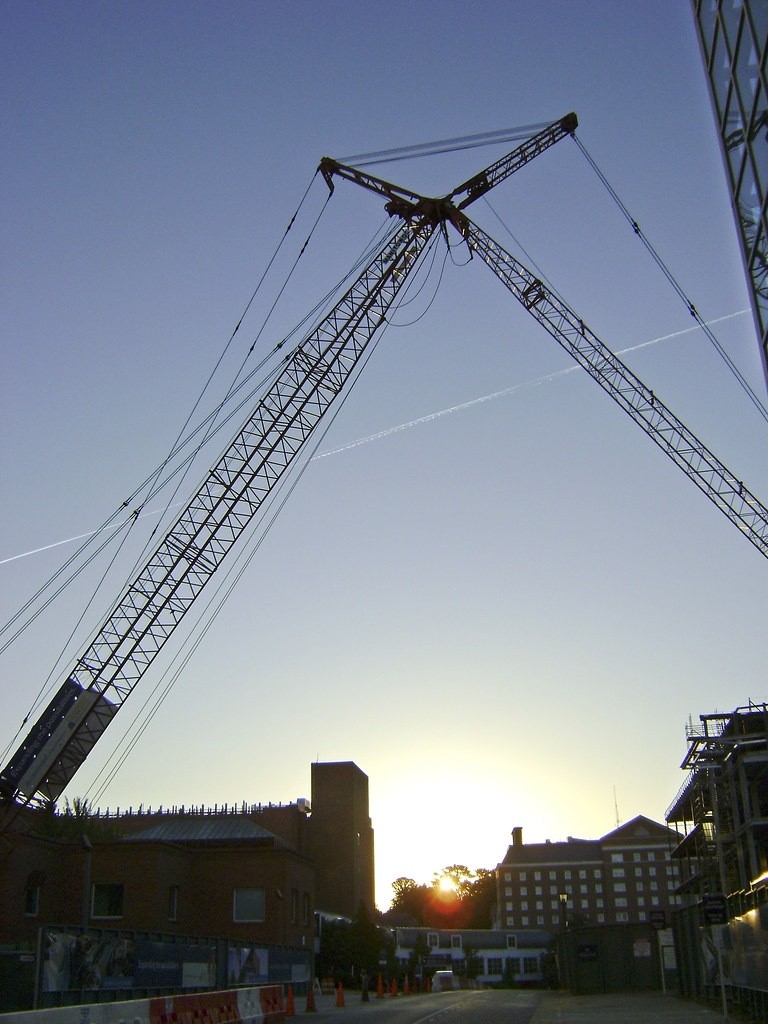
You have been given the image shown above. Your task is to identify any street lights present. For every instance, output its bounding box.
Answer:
[557,888,572,991]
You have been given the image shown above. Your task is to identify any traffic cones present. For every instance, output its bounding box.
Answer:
[402,973,411,996]
[284,984,297,1018]
[423,976,432,993]
[334,979,345,1009]
[375,975,385,997]
[302,981,317,1013]
[389,976,399,996]
[411,974,419,994]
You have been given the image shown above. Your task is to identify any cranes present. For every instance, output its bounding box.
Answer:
[0,111,768,907]
